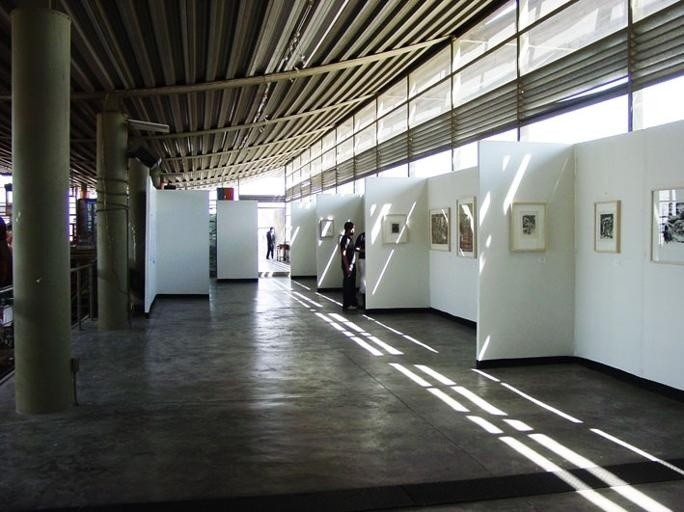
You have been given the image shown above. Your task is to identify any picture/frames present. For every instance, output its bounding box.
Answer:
[429,207,452,253]
[510,201,549,252]
[593,200,622,254]
[456,196,477,258]
[650,186,683,265]
[383,214,408,245]
[320,219,335,238]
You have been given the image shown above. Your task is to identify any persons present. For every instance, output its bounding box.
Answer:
[267,226,276,260]
[349,231,365,307]
[340,222,361,312]
